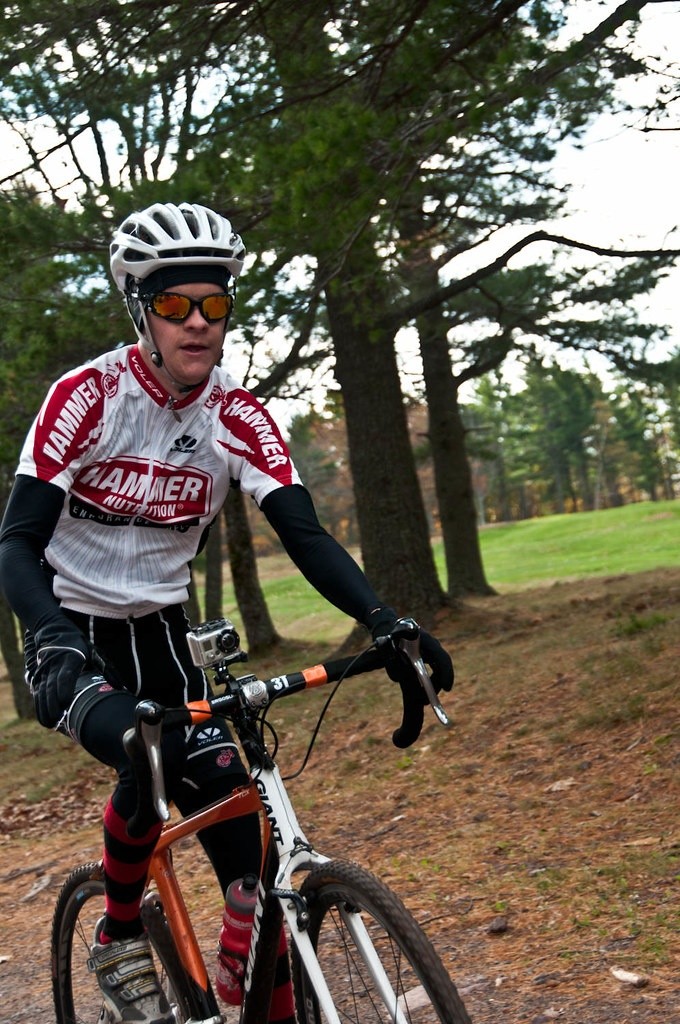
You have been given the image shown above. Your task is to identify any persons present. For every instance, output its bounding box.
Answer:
[4,203,456,1024]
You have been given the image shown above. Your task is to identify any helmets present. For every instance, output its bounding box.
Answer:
[109,201,248,333]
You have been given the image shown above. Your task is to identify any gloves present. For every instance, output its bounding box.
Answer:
[371,607,455,704]
[32,619,125,729]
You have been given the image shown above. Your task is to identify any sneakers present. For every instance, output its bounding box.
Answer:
[86,911,176,1023]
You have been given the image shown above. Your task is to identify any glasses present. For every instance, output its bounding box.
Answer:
[138,291,236,323]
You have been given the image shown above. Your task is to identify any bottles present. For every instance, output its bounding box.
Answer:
[216,876,260,1006]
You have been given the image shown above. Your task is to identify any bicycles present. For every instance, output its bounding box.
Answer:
[47,613,471,1023]
